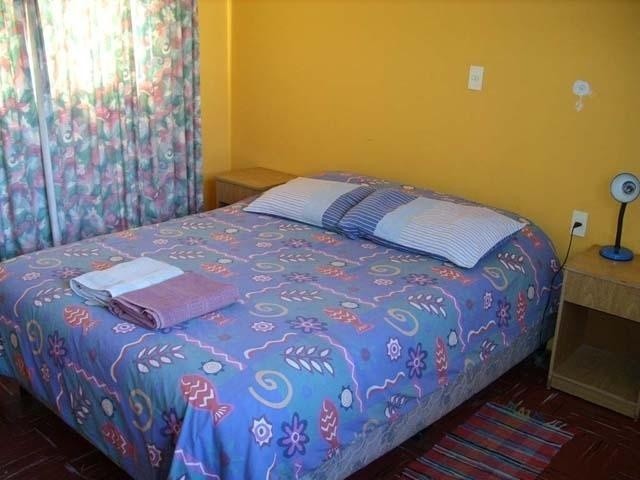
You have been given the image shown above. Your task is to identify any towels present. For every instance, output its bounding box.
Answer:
[67,255,186,308]
[105,272,242,334]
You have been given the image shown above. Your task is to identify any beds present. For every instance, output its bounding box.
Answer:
[1,164,564,480]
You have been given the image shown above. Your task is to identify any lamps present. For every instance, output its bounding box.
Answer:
[596,170,639,263]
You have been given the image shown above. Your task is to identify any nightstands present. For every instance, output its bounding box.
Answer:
[544,242,640,425]
[212,166,302,214]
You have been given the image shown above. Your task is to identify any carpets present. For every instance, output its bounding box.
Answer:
[394,400,578,480]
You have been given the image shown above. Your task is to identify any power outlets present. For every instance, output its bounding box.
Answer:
[568,207,589,238]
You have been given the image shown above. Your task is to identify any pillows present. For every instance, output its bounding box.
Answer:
[334,188,529,272]
[240,174,376,241]
